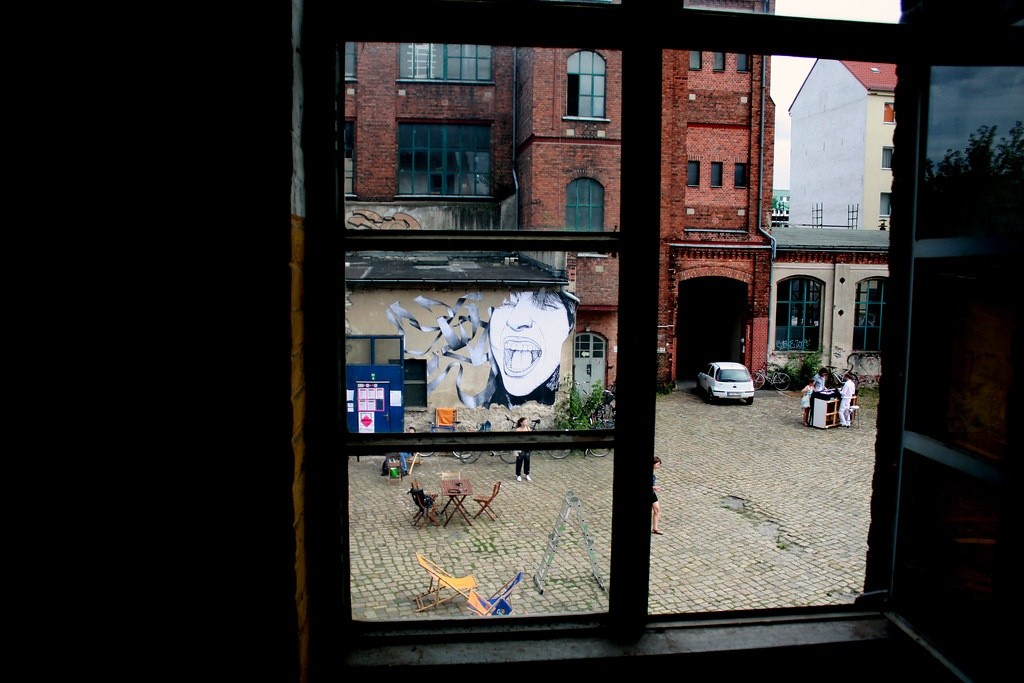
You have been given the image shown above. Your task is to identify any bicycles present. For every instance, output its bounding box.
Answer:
[751,362,790,391]
[459,428,516,465]
[579,386,616,428]
[504,414,549,455]
[547,419,611,459]
[825,365,860,389]
[418,421,473,459]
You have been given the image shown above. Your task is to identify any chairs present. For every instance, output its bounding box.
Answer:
[440,471,461,505]
[387,454,403,486]
[413,551,478,612]
[472,481,501,521]
[466,568,525,616]
[412,493,440,530]
[412,477,439,520]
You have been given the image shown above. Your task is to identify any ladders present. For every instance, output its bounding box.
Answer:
[532,491,605,594]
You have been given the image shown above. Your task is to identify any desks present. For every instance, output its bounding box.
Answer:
[441,479,473,528]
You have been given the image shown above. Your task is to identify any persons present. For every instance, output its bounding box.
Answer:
[801,379,817,425]
[512,417,533,482]
[811,368,828,392]
[837,373,855,429]
[381,427,416,477]
[651,457,663,535]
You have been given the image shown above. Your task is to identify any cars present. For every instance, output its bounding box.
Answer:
[697,362,754,405]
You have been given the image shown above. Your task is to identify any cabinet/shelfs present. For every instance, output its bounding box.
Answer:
[813,394,858,429]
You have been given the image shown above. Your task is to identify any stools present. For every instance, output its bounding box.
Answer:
[850,405,861,429]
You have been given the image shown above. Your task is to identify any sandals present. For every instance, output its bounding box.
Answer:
[652,529,662,535]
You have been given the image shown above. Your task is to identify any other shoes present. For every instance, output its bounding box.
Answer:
[402,470,407,476]
[523,474,531,480]
[801,420,805,424]
[805,422,809,426]
[836,424,849,429]
[516,475,521,481]
[381,471,389,476]
